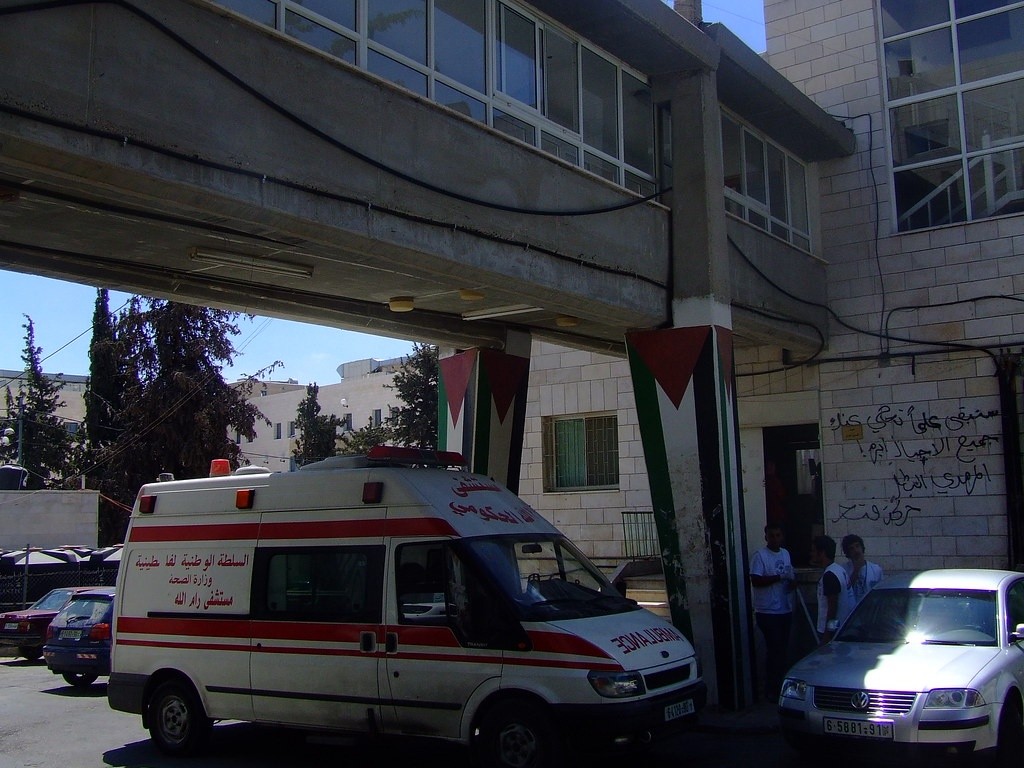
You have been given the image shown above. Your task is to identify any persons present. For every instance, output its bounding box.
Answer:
[810,535,856,649]
[749,522,800,702]
[836,533,884,624]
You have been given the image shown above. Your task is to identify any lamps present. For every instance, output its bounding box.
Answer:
[389,296,413,312]
[463,305,542,321]
[185,247,313,280]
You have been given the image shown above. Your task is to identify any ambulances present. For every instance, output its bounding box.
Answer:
[108,447,708,768]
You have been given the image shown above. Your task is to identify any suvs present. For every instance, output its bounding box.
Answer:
[43,588,115,687]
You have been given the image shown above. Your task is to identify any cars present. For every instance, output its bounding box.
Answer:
[780,569,1024,767]
[0,586,113,660]
